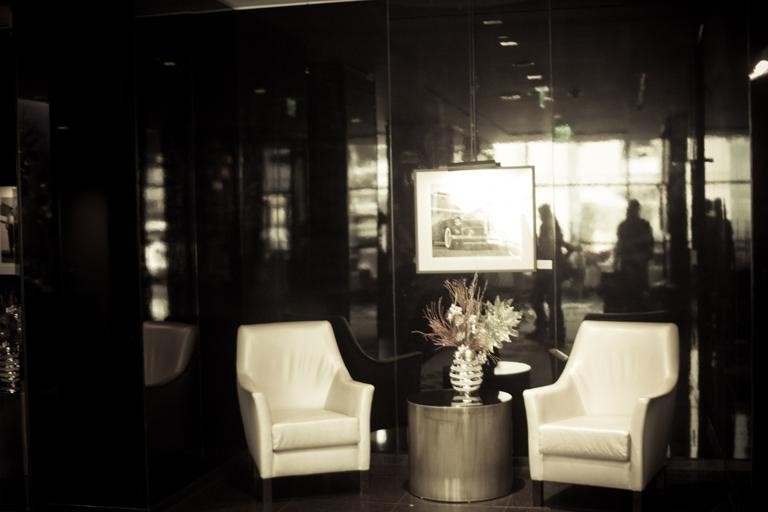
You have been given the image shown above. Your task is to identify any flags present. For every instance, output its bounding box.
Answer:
[448,361,484,401]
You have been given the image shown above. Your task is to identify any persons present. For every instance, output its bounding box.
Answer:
[614,198,656,312]
[523,203,567,342]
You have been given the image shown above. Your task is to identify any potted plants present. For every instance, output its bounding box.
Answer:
[413,166,539,273]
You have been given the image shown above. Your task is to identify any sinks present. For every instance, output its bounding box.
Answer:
[522,319,682,511]
[143,322,195,387]
[324,315,422,434]
[545,307,670,386]
[232,318,375,510]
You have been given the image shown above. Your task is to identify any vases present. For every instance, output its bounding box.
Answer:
[408,273,526,368]
